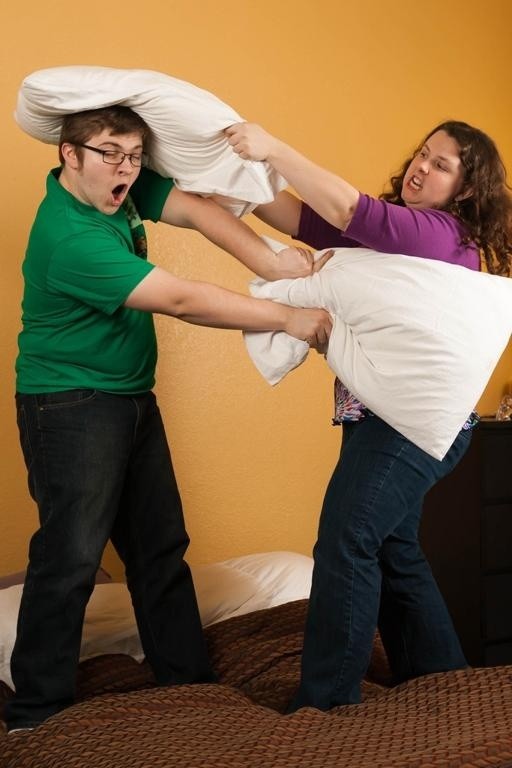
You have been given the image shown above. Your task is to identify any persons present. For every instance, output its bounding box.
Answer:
[224,120,511,714]
[10,105,335,729]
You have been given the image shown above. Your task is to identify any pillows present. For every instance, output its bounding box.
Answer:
[7,58,294,221]
[1,570,255,690]
[233,235,510,467]
[216,551,320,624]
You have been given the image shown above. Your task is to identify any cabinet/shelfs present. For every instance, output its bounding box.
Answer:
[411,415,511,672]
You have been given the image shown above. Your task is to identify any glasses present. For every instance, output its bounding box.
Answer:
[78,142,149,169]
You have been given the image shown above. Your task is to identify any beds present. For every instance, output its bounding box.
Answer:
[0,547,511,768]
